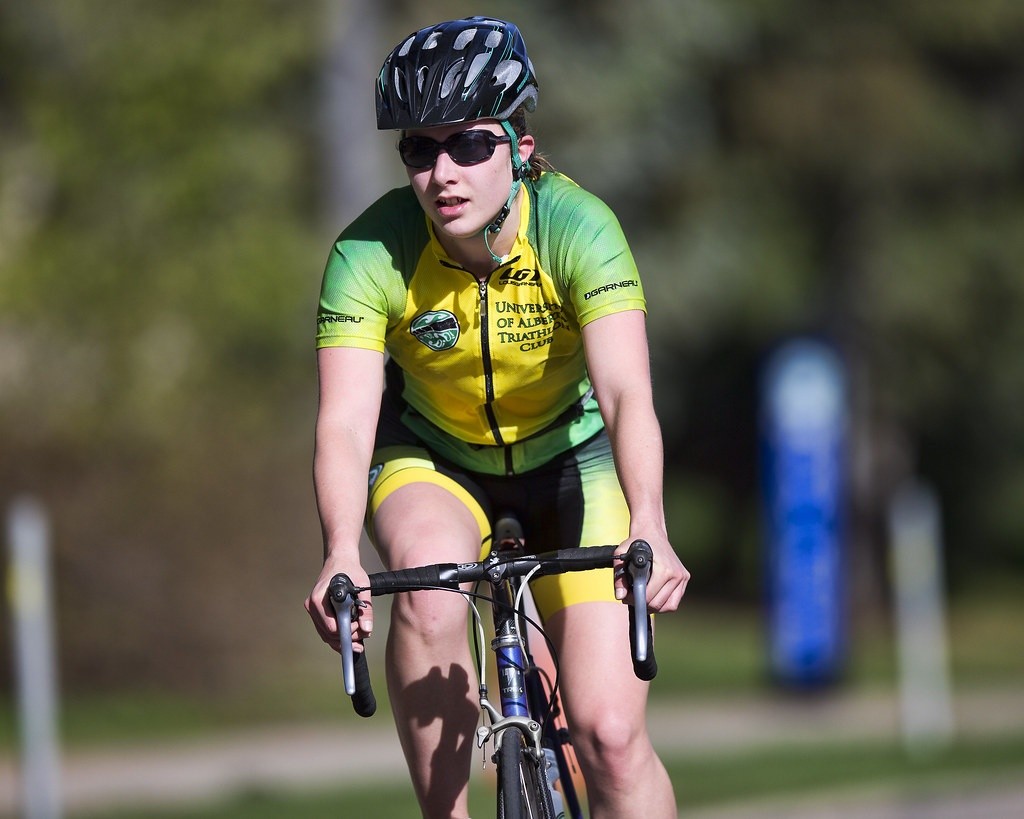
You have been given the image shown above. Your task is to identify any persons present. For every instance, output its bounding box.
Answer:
[304,18,691,818]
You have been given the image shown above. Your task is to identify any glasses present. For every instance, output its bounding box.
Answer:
[395,128,511,169]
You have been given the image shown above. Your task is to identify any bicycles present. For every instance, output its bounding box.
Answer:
[328,536,658,818]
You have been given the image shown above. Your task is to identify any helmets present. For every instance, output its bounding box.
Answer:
[374,15,539,129]
